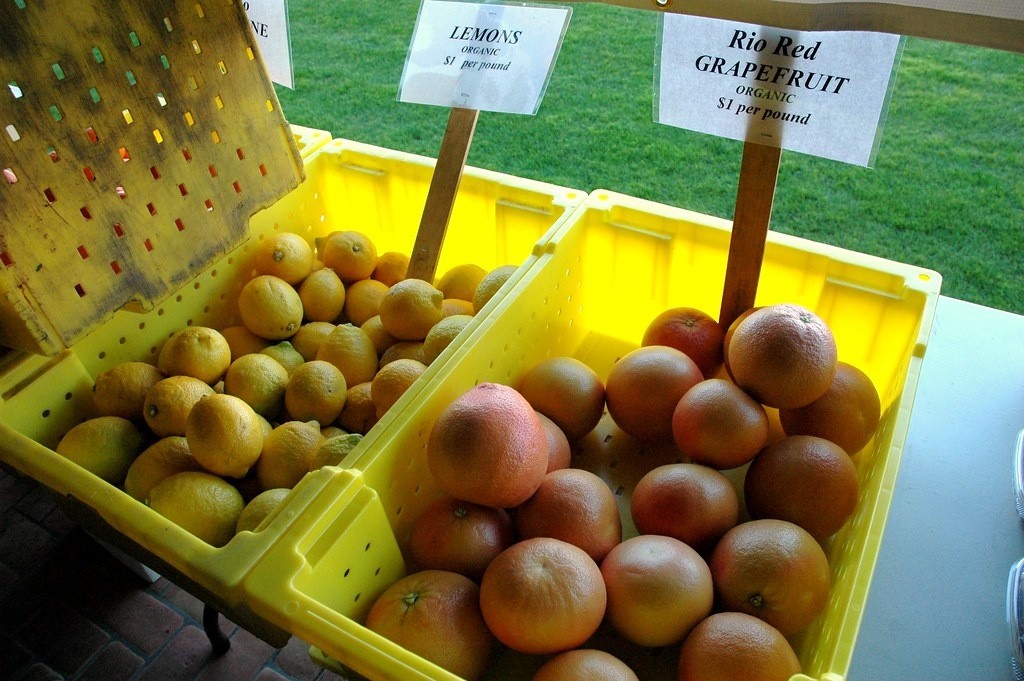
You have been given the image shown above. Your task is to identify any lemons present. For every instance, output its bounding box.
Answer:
[50,231,519,547]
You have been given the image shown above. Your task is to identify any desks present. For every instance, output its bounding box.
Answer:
[61,288,1024,681]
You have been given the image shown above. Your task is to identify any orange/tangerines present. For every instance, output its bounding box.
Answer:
[367,305,881,681]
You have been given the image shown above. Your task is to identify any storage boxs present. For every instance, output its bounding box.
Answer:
[0,111,943,681]
